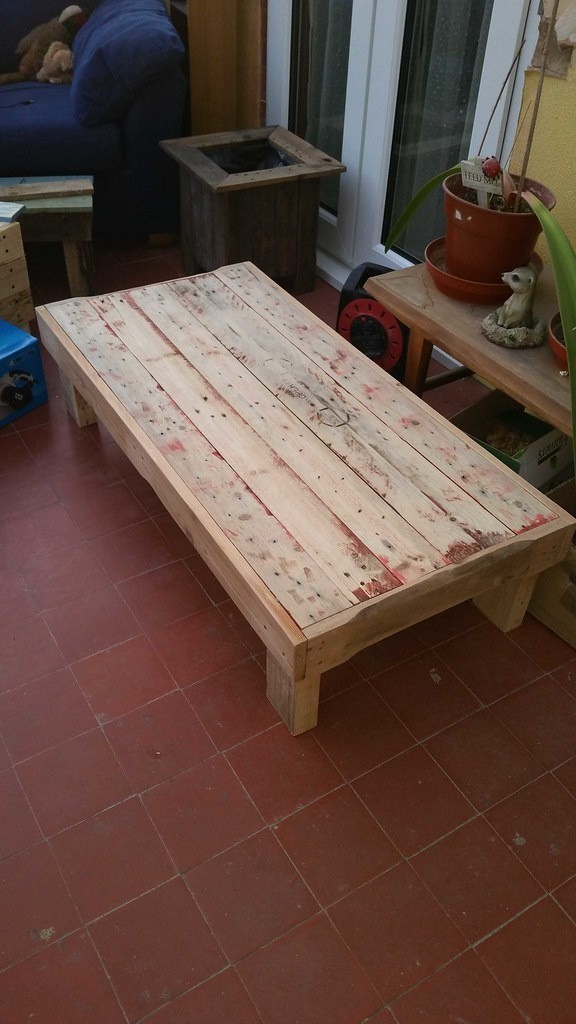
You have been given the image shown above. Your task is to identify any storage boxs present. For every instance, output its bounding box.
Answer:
[0,220,25,266]
[0,315,49,429]
[524,476,576,651]
[0,253,30,302]
[0,287,35,324]
[441,387,573,496]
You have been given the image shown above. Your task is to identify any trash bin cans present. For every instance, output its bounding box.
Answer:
[159,125,345,292]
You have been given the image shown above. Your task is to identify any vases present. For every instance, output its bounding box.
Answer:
[153,123,348,293]
[547,309,569,365]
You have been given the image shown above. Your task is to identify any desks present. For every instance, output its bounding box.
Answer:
[30,255,576,738]
[364,258,576,439]
[0,173,103,299]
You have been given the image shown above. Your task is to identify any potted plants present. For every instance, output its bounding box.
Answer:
[383,149,576,445]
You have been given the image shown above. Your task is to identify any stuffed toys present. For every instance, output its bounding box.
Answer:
[36,42,74,85]
[13,5,89,80]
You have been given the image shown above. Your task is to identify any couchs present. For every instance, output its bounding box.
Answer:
[0,0,184,252]
